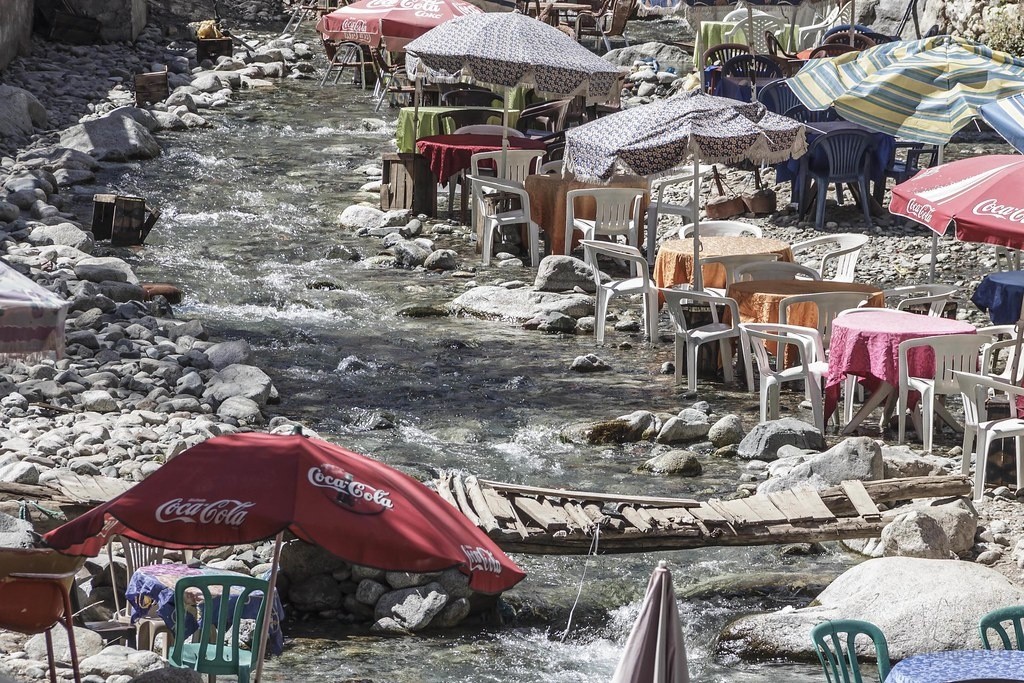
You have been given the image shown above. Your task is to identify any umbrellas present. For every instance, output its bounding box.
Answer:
[786,35,1024,387]
[44,432,526,683]
[606,561,694,683]
[402,13,630,233]
[561,89,809,303]
[315,0,486,142]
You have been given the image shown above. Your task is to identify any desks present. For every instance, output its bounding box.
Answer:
[716,77,791,103]
[125,565,284,683]
[692,20,800,72]
[882,648,1024,683]
[524,3,592,38]
[824,311,977,439]
[653,236,795,312]
[775,121,896,210]
[969,271,1024,376]
[521,171,649,255]
[394,104,520,176]
[715,280,884,382]
[416,134,548,212]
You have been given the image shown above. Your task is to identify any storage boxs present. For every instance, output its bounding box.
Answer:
[91,193,162,247]
[197,35,232,63]
[135,65,169,106]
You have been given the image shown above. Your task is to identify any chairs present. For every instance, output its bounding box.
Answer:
[284,0,1024,512]
[811,620,891,683]
[979,604,1024,652]
[1,533,270,683]
